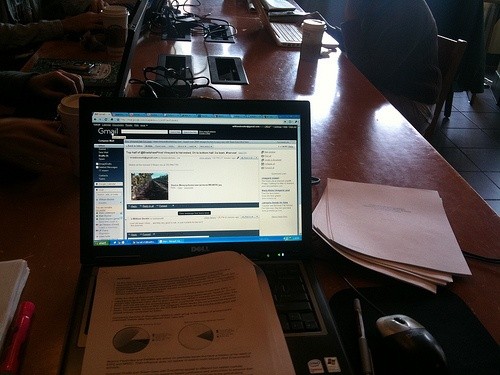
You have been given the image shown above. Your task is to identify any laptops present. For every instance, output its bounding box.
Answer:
[62,96,352,375]
[252,0,340,48]
[34,0,148,95]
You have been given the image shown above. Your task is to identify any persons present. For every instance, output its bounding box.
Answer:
[0,0,110,72]
[0,70,83,150]
[296,0,442,136]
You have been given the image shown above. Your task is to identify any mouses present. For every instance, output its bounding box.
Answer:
[376,315,446,375]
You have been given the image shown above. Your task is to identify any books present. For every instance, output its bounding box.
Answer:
[311,177,473,293]
[80,251,295,375]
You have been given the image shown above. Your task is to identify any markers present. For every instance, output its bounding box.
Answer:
[0,301,35,375]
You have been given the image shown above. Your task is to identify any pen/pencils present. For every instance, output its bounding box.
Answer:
[354,297,373,375]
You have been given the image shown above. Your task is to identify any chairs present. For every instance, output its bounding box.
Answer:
[418,34,469,143]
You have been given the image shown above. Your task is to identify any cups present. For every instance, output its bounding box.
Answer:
[101,6,130,56]
[57,93,99,167]
[301,19,327,61]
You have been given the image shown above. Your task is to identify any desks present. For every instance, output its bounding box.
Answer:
[0,0,500,375]
